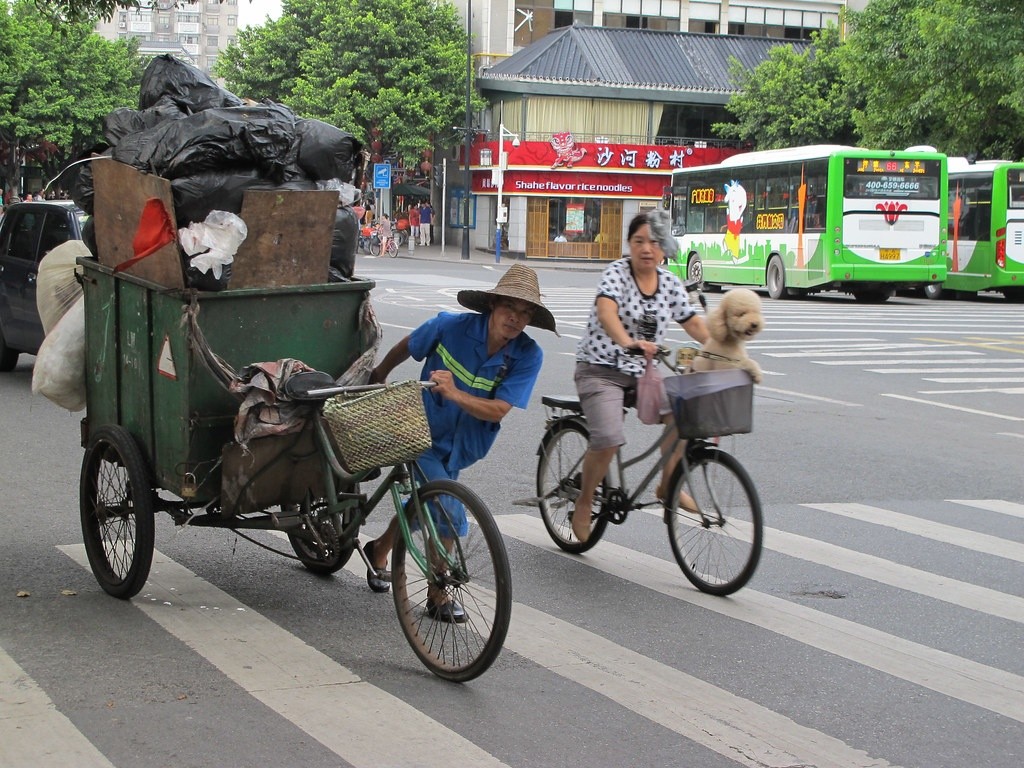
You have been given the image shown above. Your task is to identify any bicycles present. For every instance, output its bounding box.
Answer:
[358,220,409,258]
[511,336,765,597]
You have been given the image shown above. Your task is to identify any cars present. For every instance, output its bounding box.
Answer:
[0,197,92,376]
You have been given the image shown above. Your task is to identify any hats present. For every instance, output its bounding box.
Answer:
[458,264,556,332]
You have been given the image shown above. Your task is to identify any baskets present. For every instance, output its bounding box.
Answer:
[323,379,433,472]
[663,368,753,439]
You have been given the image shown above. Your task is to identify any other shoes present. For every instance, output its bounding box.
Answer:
[363,540,390,592]
[420,244,425,246]
[657,485,700,514]
[427,243,430,246]
[427,599,468,623]
[572,498,590,543]
[378,255,384,258]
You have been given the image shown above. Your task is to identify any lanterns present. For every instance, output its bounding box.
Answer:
[422,161,432,175]
[422,149,432,161]
[371,153,382,163]
[371,140,382,151]
[372,127,382,137]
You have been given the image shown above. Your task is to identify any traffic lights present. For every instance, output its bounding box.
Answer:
[433,164,444,188]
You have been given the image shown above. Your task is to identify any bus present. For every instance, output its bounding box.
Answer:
[661,144,949,304]
[944,155,1023,303]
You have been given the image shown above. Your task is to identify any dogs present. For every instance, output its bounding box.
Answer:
[681,288,764,385]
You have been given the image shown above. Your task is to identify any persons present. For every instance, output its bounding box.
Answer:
[354,198,376,230]
[361,266,555,623]
[378,213,392,258]
[570,212,709,544]
[0,188,46,223]
[406,198,436,247]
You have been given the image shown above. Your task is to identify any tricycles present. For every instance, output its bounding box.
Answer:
[77,257,513,683]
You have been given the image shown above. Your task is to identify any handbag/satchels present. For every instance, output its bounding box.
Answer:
[637,353,662,424]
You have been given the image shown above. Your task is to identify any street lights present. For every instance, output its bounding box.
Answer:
[494,124,521,265]
